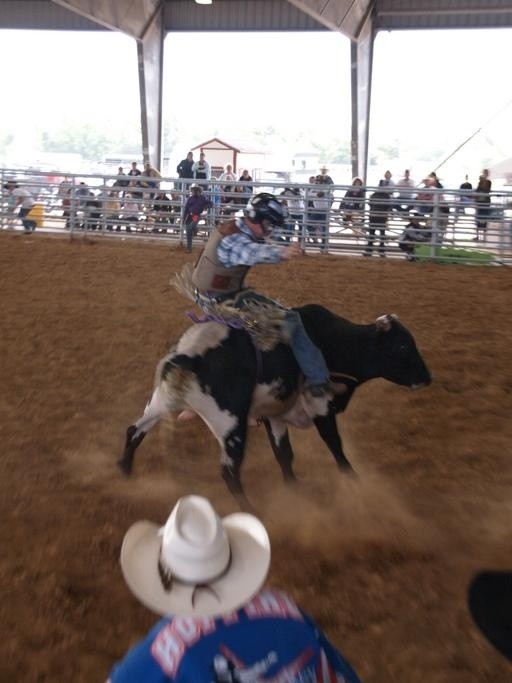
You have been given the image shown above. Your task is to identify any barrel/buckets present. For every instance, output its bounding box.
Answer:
[26,201,44,227]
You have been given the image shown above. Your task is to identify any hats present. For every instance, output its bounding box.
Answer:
[119,493,272,619]
[189,183,203,192]
[318,165,331,171]
[242,191,286,228]
[421,175,435,182]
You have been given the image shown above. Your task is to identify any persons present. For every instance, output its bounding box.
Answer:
[173,197,348,397]
[4,155,493,263]
[105,494,359,683]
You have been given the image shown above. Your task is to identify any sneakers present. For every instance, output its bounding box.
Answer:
[311,377,349,399]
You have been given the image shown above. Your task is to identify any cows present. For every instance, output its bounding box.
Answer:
[114,301,433,522]
